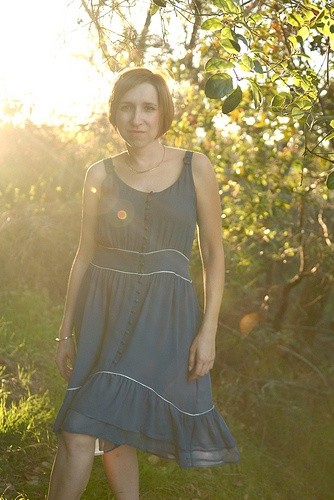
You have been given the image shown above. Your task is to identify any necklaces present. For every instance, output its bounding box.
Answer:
[123,145,166,174]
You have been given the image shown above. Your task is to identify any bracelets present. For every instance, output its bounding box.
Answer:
[55,334,73,343]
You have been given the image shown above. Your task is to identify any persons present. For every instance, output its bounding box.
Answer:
[45,67,245,500]
